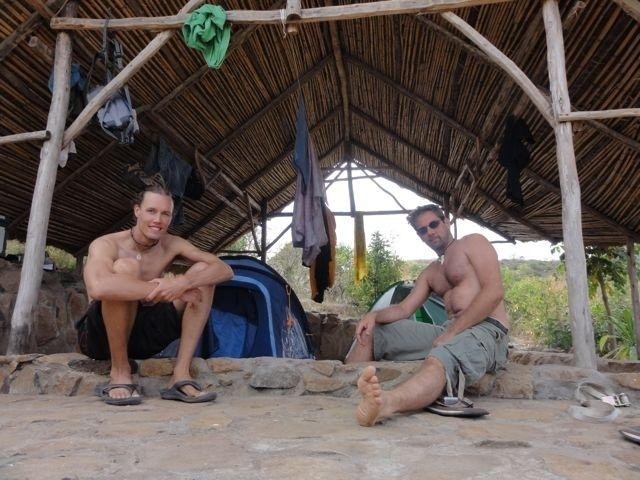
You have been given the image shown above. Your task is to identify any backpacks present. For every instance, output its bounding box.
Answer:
[85,18,139,146]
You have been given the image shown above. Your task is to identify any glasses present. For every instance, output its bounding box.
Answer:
[418,219,441,236]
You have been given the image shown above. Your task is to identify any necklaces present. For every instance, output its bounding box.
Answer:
[438,239,455,265]
[130,226,160,261]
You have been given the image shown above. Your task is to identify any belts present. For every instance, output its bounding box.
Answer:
[570,381,631,423]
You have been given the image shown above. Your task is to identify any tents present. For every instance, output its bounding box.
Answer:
[338,278,447,363]
[157,253,317,361]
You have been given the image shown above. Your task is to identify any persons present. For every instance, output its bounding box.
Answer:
[74,184,234,406]
[342,205,511,428]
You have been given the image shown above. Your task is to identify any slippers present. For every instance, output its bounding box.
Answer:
[160,380,216,403]
[619,426,640,442]
[426,398,487,417]
[101,383,142,405]
